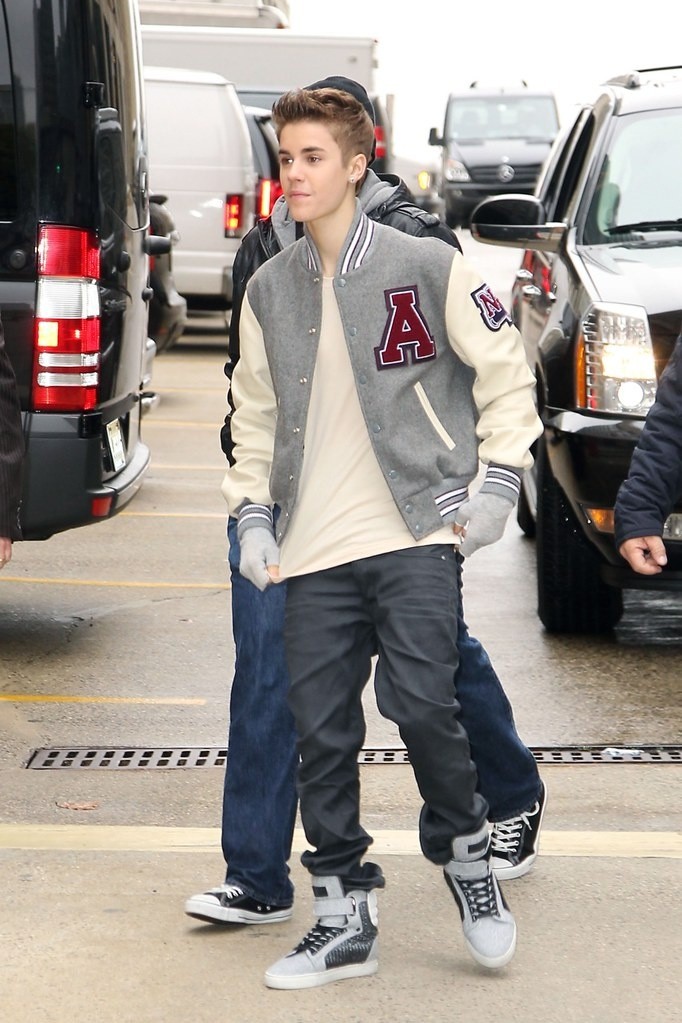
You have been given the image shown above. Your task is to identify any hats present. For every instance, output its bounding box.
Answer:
[303,75,377,123]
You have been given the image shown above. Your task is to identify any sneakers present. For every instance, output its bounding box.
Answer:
[263,876,382,988]
[185,886,294,925]
[445,860,517,967]
[487,781,546,875]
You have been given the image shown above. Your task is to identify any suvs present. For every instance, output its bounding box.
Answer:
[468,66,682,636]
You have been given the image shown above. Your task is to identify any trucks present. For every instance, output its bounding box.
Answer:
[428,81,570,226]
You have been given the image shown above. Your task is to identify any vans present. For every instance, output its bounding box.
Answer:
[140,64,255,312]
[0,0,168,542]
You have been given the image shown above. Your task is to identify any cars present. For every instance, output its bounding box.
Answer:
[147,193,186,356]
[243,106,285,222]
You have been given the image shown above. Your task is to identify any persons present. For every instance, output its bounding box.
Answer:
[185,75,548,923]
[223,87,543,989]
[613,331,682,575]
